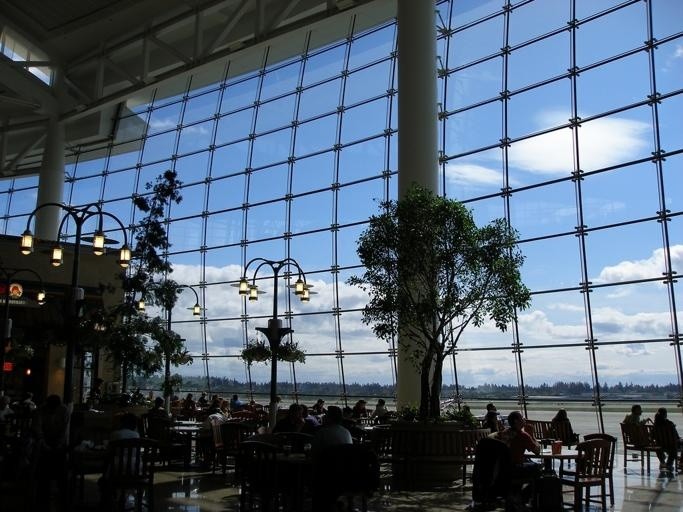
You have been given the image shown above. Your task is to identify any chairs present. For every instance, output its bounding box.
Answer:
[621,423,682,473]
[0,400,618,512]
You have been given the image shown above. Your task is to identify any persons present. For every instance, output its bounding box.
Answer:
[623,405,666,464]
[651,406,682,471]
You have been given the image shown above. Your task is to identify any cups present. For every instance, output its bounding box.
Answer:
[550,439,562,454]
[303,443,312,457]
[281,445,293,456]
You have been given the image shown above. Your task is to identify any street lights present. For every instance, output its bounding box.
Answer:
[20,203,132,404]
[139,281,207,397]
[232,258,318,426]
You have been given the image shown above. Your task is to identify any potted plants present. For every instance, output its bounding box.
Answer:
[343,177,531,484]
